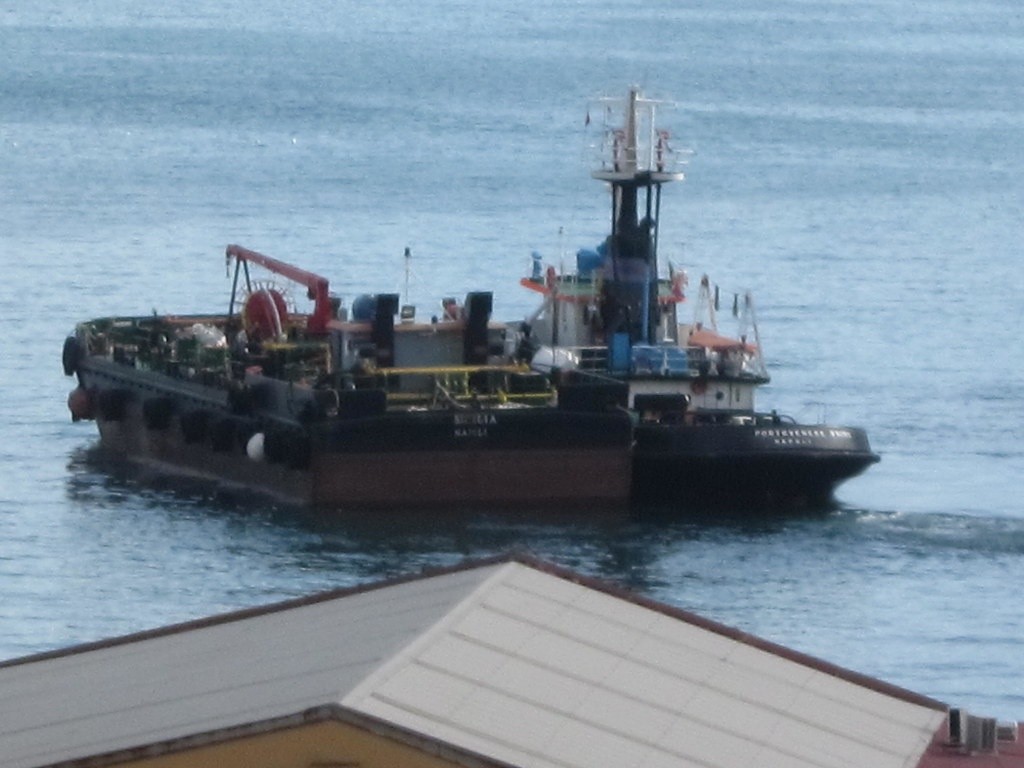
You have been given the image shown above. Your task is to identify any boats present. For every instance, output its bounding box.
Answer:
[62,83,880,515]
